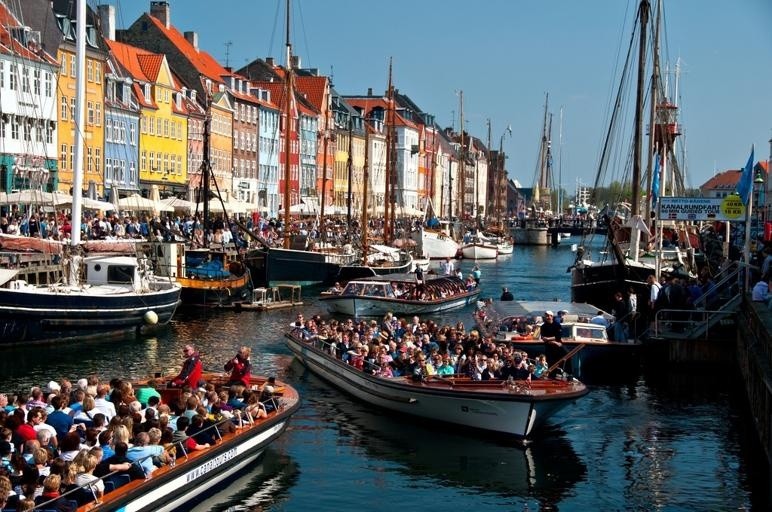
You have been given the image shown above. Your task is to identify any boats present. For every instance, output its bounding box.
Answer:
[0,370,298,512]
[280,311,594,441]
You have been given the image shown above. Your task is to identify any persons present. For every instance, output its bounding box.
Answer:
[646,209,772,321]
